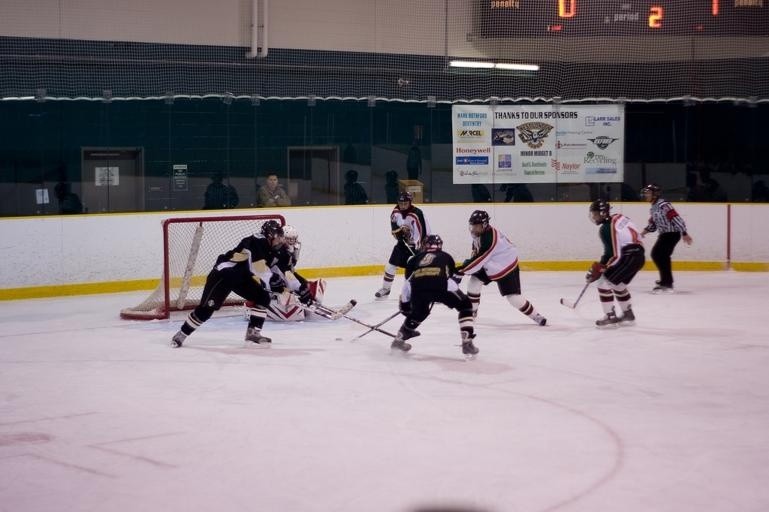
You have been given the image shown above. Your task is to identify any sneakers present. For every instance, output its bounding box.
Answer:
[533,313,546,326]
[375,288,391,297]
[654,279,672,290]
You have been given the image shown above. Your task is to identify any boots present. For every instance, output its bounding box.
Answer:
[245,326,271,343]
[392,324,420,351]
[596,305,619,325]
[460,326,479,354]
[619,304,635,322]
[172,326,189,347]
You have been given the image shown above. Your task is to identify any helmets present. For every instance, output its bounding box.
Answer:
[642,184,661,198]
[261,221,299,253]
[590,199,609,225]
[426,235,442,250]
[397,192,413,202]
[469,210,490,238]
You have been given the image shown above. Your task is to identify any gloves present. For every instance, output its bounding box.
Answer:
[452,267,465,284]
[586,262,607,283]
[396,229,408,244]
[270,272,286,294]
[295,284,317,307]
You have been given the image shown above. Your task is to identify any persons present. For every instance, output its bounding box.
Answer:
[344,170,368,205]
[54,181,83,214]
[395,235,479,357]
[172,219,311,354]
[446,209,547,328]
[373,194,427,299]
[384,171,401,203]
[687,170,727,203]
[246,225,326,320]
[586,199,645,329]
[203,174,237,207]
[257,173,291,207]
[406,142,425,179]
[473,184,492,202]
[636,184,693,292]
[500,183,536,204]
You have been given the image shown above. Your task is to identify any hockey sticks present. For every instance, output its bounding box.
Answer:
[334,311,403,342]
[560,284,589,309]
[265,289,357,320]
